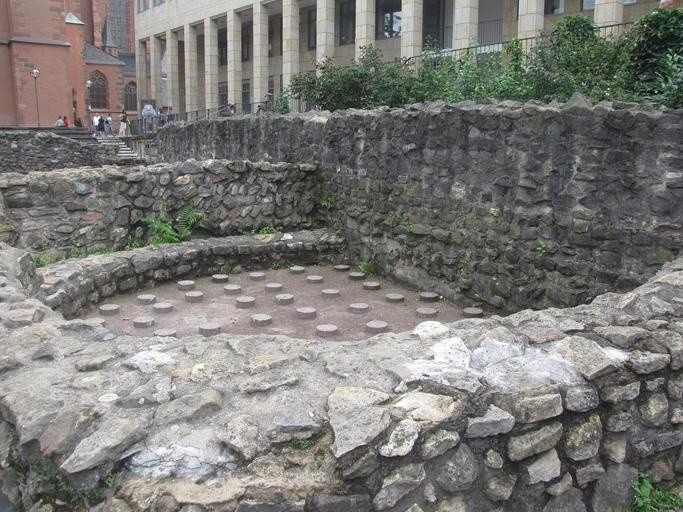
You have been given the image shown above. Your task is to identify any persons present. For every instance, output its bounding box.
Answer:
[116,110,126,136]
[62,116,68,127]
[103,113,115,136]
[97,115,104,137]
[92,113,100,136]
[54,116,62,127]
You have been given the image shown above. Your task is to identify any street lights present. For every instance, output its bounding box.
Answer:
[85,79,93,127]
[30,67,41,126]
